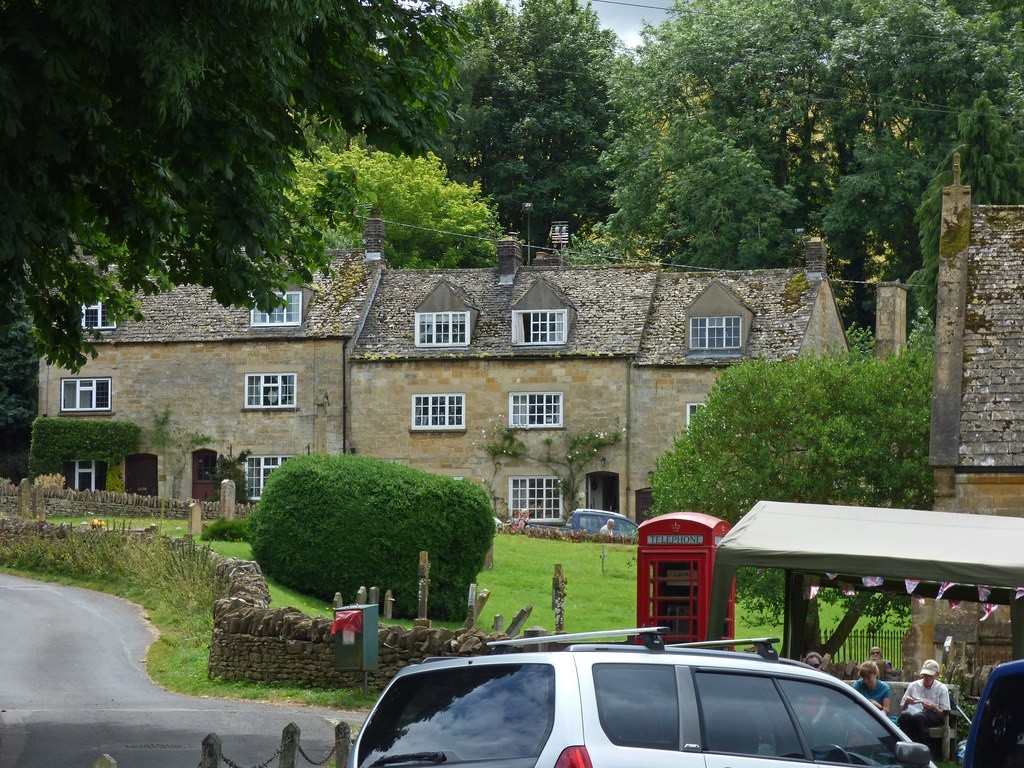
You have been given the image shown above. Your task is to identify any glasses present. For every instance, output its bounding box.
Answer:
[872,652,879,654]
[809,664,820,669]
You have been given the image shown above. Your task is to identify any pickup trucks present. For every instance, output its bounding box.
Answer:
[527,508,639,539]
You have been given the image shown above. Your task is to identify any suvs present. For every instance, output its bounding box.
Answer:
[349,626,937,768]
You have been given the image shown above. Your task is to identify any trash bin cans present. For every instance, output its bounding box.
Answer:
[334,604,379,674]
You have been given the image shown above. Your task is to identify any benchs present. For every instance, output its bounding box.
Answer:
[845,679,960,760]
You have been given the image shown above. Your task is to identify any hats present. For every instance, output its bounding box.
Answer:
[920,660,939,676]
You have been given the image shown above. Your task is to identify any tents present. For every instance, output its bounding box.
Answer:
[706,500,1024,662]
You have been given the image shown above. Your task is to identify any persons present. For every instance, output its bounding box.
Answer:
[897,658,951,749]
[517,509,531,528]
[870,646,890,669]
[806,652,823,670]
[853,661,890,716]
[600,519,615,537]
